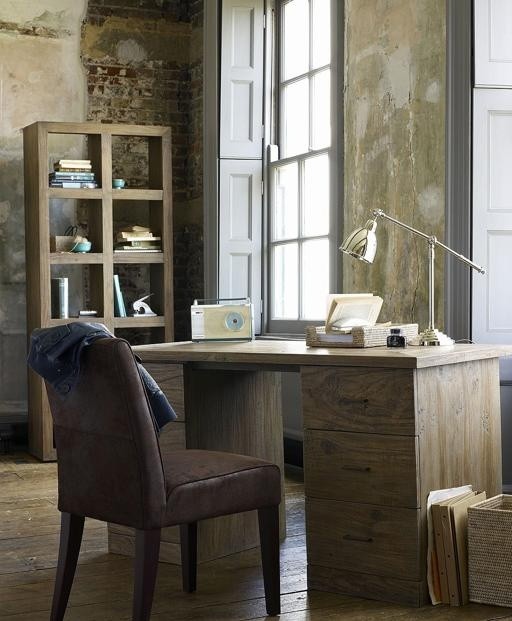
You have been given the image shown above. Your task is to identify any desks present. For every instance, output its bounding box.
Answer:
[109,341,501,609]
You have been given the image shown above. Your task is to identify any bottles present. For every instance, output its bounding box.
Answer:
[386,328,406,347]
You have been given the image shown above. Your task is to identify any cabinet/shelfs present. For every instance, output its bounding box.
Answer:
[23,120,177,465]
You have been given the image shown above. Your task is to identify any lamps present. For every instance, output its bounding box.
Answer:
[339,206,484,348]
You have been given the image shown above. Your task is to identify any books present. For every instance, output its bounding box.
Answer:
[113,225,162,253]
[48,158,99,189]
[51,277,68,319]
[113,274,127,318]
[326,292,391,333]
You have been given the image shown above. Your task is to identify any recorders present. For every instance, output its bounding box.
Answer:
[190,297,255,344]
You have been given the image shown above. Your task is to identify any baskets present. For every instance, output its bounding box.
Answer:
[467,493,512,608]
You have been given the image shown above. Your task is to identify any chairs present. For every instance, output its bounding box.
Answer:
[45,329,282,621]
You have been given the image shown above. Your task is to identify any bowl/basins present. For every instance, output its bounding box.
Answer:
[112,179,125,189]
[71,242,92,253]
